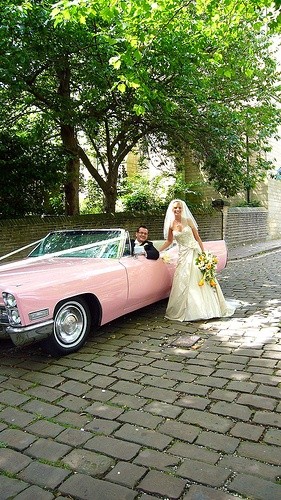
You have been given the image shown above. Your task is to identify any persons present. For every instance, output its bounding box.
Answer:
[158,199,237,322]
[123,226,159,260]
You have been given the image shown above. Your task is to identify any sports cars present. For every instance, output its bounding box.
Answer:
[0,226,230,354]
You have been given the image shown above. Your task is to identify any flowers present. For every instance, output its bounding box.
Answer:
[196,252,218,286]
